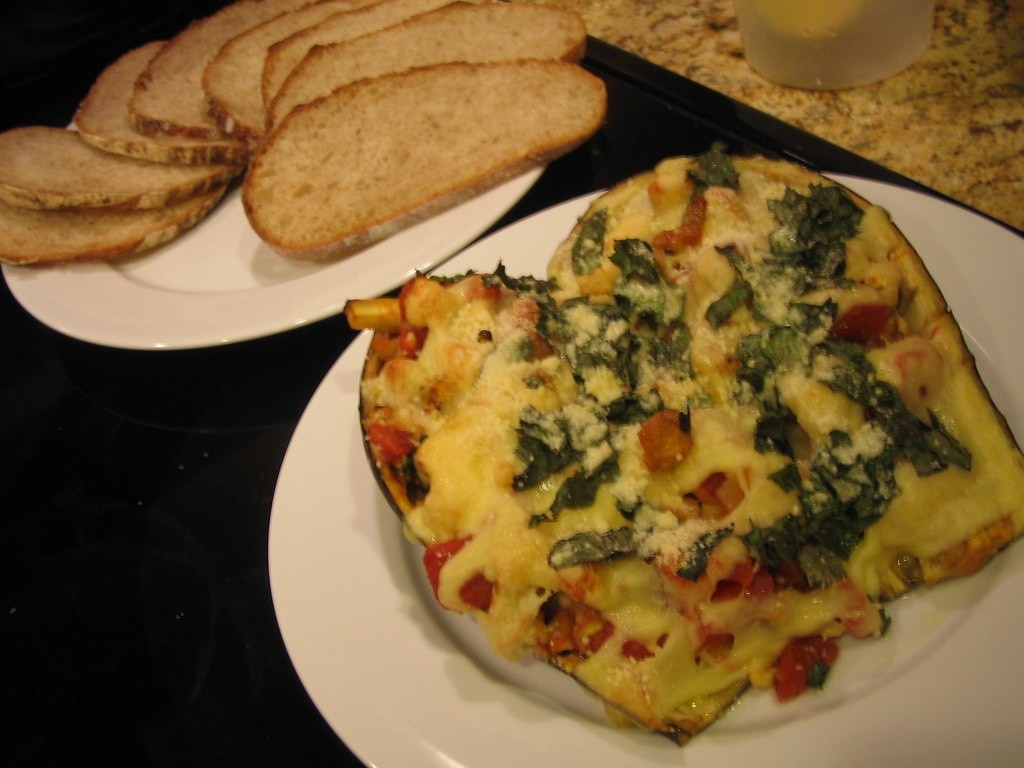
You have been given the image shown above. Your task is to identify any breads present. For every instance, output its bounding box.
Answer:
[0,0,606,268]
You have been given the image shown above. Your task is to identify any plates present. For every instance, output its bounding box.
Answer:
[2,120,548,352]
[267,174,1022,768]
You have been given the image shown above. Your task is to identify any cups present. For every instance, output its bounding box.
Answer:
[733,1,933,91]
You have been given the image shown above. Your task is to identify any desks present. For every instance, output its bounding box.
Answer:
[0,0,1024,768]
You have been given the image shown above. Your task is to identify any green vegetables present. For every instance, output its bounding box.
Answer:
[426,142,974,597]
